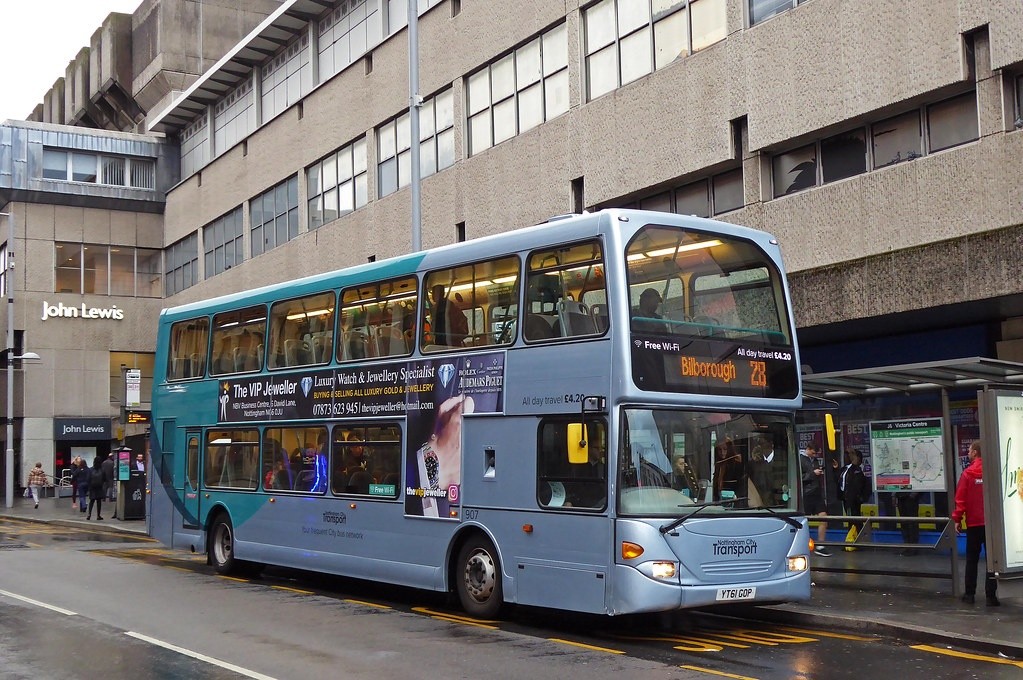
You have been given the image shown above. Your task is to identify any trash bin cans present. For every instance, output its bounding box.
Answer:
[116,470,146,521]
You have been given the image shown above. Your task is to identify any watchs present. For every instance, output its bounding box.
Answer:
[421,442,440,491]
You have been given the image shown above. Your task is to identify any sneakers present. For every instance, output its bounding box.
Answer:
[813,547,834,557]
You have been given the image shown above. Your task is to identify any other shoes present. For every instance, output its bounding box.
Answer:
[986,595,1001,606]
[35,504,38,509]
[87,514,91,520]
[97,516,103,520]
[72,502,78,508]
[83,506,87,512]
[903,549,918,555]
[962,592,975,603]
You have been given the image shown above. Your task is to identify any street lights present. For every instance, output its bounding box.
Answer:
[6,352,41,512]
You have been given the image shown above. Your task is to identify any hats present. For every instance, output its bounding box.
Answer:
[306,443,314,449]
[108,453,114,457]
[640,288,663,302]
[36,462,42,466]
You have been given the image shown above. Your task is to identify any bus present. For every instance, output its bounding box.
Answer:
[141,208,815,621]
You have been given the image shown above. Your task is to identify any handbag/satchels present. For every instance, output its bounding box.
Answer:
[23,487,30,497]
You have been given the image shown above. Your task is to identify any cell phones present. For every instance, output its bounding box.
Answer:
[820,467,826,470]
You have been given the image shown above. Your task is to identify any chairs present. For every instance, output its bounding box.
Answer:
[188,434,393,494]
[175,300,727,376]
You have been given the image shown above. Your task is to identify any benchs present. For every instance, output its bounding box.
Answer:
[812,519,957,551]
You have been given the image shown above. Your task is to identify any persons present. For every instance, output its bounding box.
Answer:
[261,428,378,495]
[131,452,146,471]
[70,452,117,520]
[890,492,924,557]
[748,434,789,509]
[799,440,834,557]
[630,288,668,333]
[430,284,462,347]
[672,455,698,498]
[951,438,1002,607]
[407,354,504,522]
[713,437,746,510]
[563,435,607,507]
[831,447,865,534]
[27,462,51,510]
